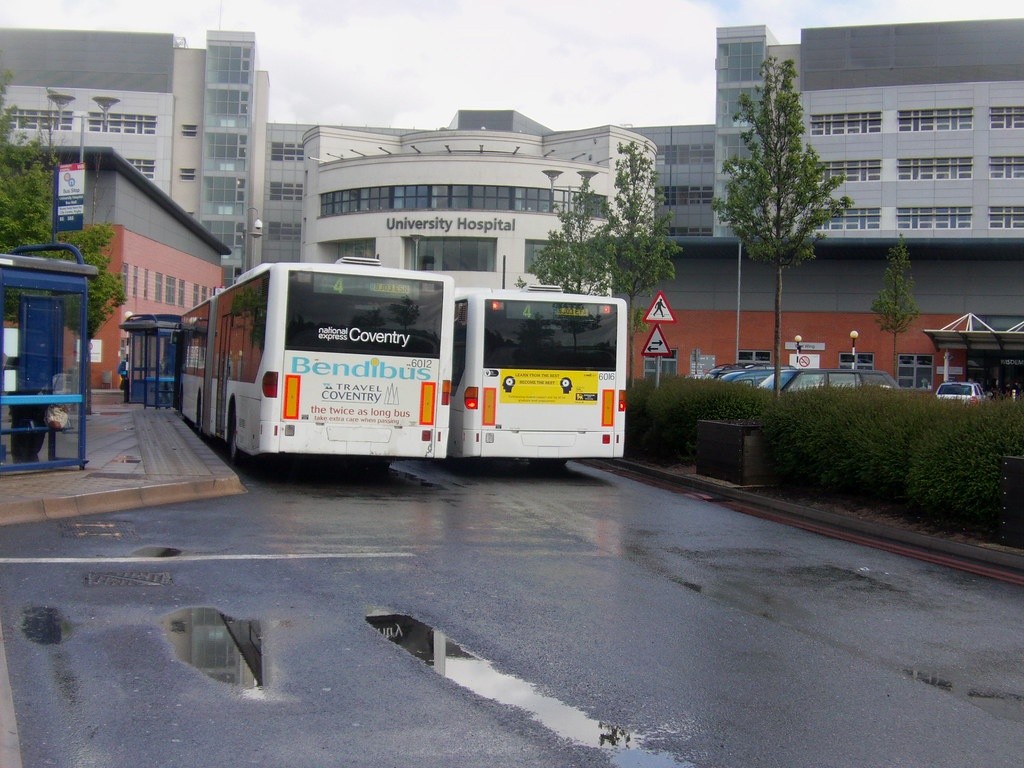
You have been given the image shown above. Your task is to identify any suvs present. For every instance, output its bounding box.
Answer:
[704,362,901,394]
[929,380,993,406]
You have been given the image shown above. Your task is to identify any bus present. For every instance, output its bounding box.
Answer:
[442,284,628,476]
[179,257,456,484]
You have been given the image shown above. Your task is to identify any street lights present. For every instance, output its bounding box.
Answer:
[794,335,803,372]
[232,208,262,284]
[74,110,89,162]
[410,234,424,302]
[249,230,263,268]
[850,329,858,369]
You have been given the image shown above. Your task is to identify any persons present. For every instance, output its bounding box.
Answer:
[968,378,1024,396]
[118,354,128,403]
[4,357,74,463]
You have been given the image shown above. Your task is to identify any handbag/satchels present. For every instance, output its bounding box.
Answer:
[45,404,68,429]
[118,360,127,375]
